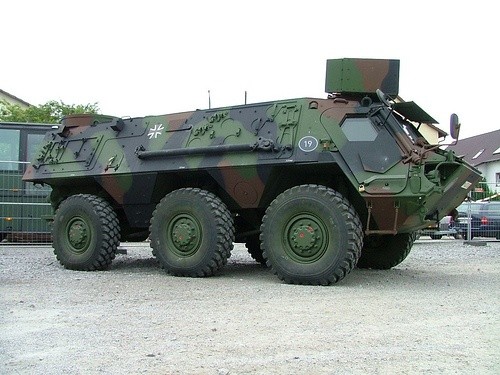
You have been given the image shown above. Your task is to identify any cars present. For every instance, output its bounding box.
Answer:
[456,200,500,240]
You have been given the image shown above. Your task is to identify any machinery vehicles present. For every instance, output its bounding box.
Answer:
[26,58,487,286]
